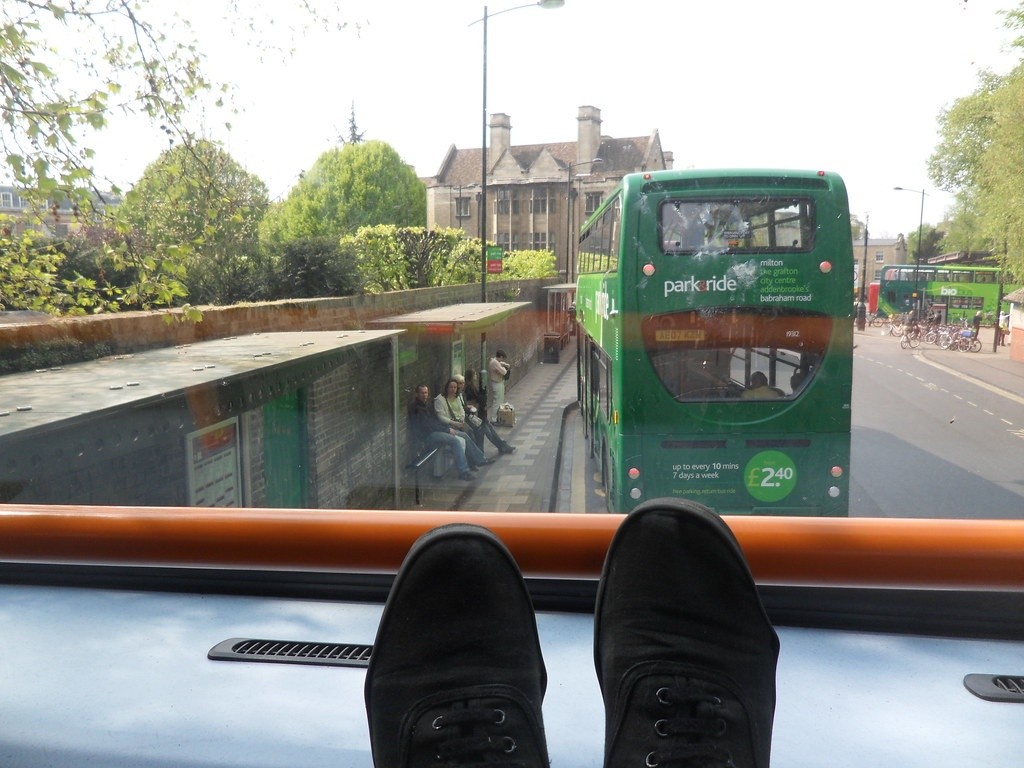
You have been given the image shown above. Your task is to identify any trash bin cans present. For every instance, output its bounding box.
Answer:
[543,332,560,363]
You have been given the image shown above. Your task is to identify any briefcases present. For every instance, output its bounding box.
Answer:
[498,402,515,427]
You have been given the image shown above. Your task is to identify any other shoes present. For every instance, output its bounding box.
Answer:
[477,457,496,467]
[459,471,475,481]
[501,444,518,454]
[470,464,479,471]
[498,447,503,455]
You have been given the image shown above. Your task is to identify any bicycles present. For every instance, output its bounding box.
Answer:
[853,309,982,353]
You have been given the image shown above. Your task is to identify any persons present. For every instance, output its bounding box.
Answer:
[409,385,496,481]
[364,497,780,768]
[973,311,982,340]
[927,309,941,325]
[451,369,518,455]
[743,371,803,398]
[433,380,480,472]
[904,310,915,340]
[998,310,1009,346]
[569,302,576,336]
[488,349,510,425]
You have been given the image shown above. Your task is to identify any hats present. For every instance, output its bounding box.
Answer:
[497,349,507,358]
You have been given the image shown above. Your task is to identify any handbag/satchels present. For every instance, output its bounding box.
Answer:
[460,423,477,441]
[467,414,483,429]
[504,366,510,380]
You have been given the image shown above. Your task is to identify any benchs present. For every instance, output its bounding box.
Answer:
[405,442,443,505]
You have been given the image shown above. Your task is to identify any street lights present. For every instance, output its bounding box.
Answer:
[894,187,925,310]
[479,1,569,420]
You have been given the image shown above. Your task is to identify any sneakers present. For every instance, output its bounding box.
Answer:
[364,524,549,768]
[593,498,780,768]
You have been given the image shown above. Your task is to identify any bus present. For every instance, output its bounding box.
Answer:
[568,167,853,523]
[878,265,1024,328]
